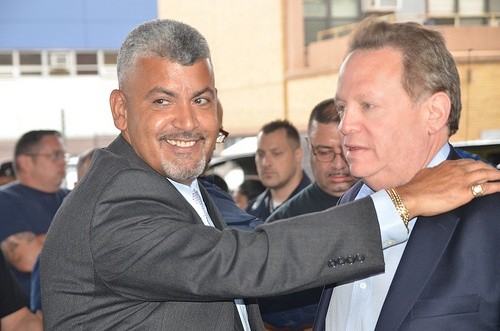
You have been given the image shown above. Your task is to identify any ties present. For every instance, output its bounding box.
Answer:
[191,188,251,331]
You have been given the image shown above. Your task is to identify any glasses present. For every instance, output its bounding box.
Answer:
[23,149,71,162]
[309,137,348,163]
[216,129,229,145]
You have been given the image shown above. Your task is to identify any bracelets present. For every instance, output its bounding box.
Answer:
[384,185,410,228]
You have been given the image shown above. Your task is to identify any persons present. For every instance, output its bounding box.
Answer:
[0,129,74,304]
[199,99,323,331]
[233,179,265,213]
[310,22,499,330]
[0,161,17,188]
[0,249,43,331]
[244,118,315,221]
[38,19,500,331]
[76,148,102,181]
[264,98,363,225]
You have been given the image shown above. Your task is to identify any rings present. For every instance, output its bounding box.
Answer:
[471,183,484,198]
